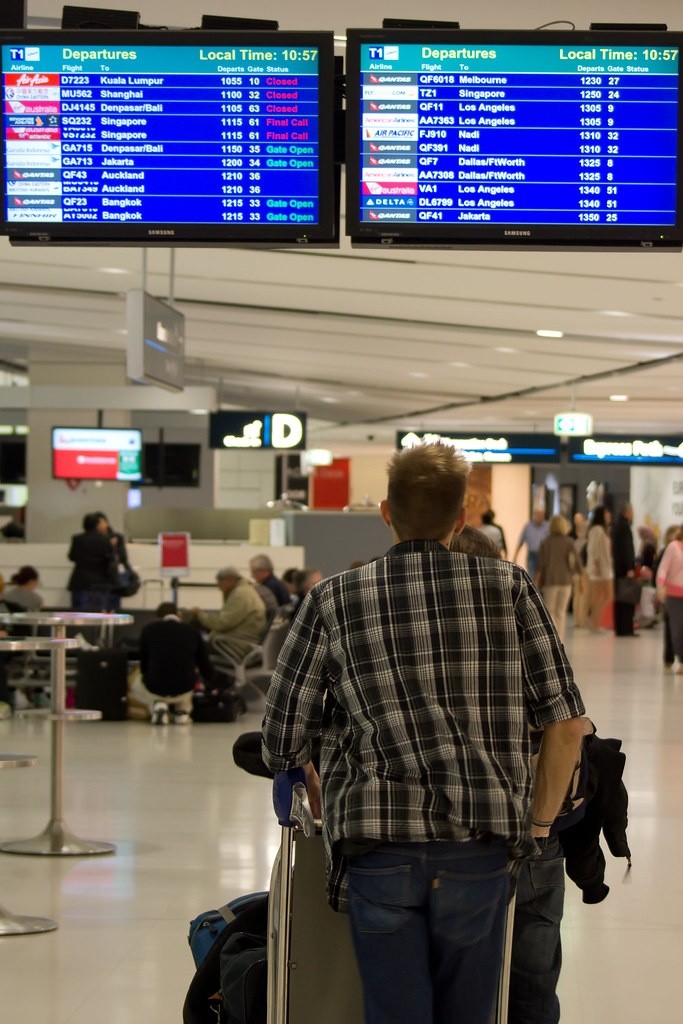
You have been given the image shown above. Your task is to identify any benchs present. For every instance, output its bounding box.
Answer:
[7,602,121,707]
[210,619,292,702]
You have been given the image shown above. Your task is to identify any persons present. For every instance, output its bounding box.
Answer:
[131,602,218,725]
[261,443,586,1024]
[512,506,589,642]
[66,511,127,643]
[636,527,659,628]
[651,522,683,674]
[0,566,43,719]
[609,503,641,637]
[192,567,266,696]
[450,525,592,1024]
[449,510,507,559]
[249,555,321,623]
[586,506,613,635]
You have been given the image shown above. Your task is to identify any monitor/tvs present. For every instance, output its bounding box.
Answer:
[347,26,683,248]
[51,427,143,482]
[131,442,200,487]
[0,30,340,244]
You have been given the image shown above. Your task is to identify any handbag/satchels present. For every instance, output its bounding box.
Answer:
[183,890,268,1024]
[118,568,140,598]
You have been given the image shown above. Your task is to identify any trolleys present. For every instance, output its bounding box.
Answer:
[262,769,532,1024]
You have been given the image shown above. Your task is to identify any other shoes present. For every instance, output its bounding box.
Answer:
[175,714,190,725]
[151,702,167,725]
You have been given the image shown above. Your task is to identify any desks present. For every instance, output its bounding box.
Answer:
[0,608,136,861]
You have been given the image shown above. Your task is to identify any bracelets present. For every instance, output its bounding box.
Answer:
[532,818,553,827]
